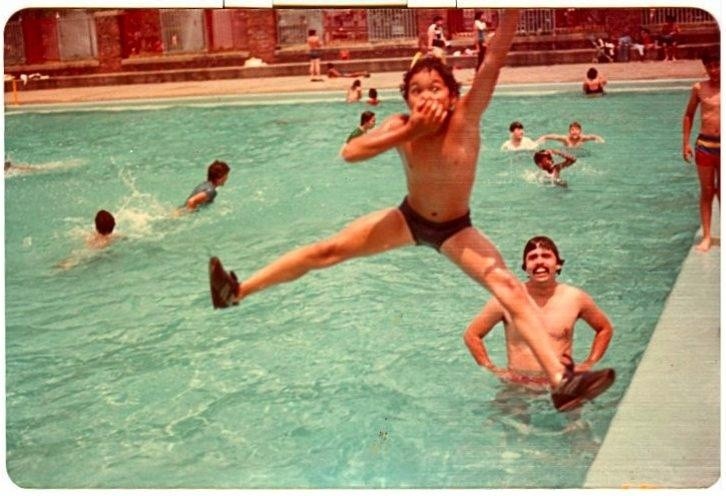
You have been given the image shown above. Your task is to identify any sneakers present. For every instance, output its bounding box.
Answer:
[209,258,241,311]
[552,355,614,414]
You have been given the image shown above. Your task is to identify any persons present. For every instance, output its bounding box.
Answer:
[347,111,376,142]
[409,15,452,71]
[464,237,613,386]
[682,50,720,251]
[209,7,617,413]
[87,210,121,249]
[583,67,608,95]
[473,10,489,72]
[307,12,381,105]
[4,72,41,90]
[501,122,606,182]
[592,14,682,64]
[176,160,230,212]
